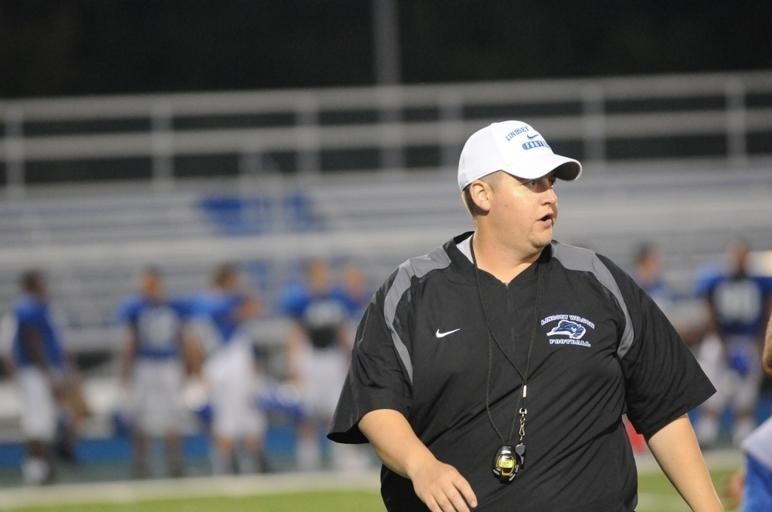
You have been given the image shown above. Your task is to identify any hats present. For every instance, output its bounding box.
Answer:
[457,120,582,192]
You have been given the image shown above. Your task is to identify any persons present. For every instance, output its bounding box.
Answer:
[325,118,723,511]
